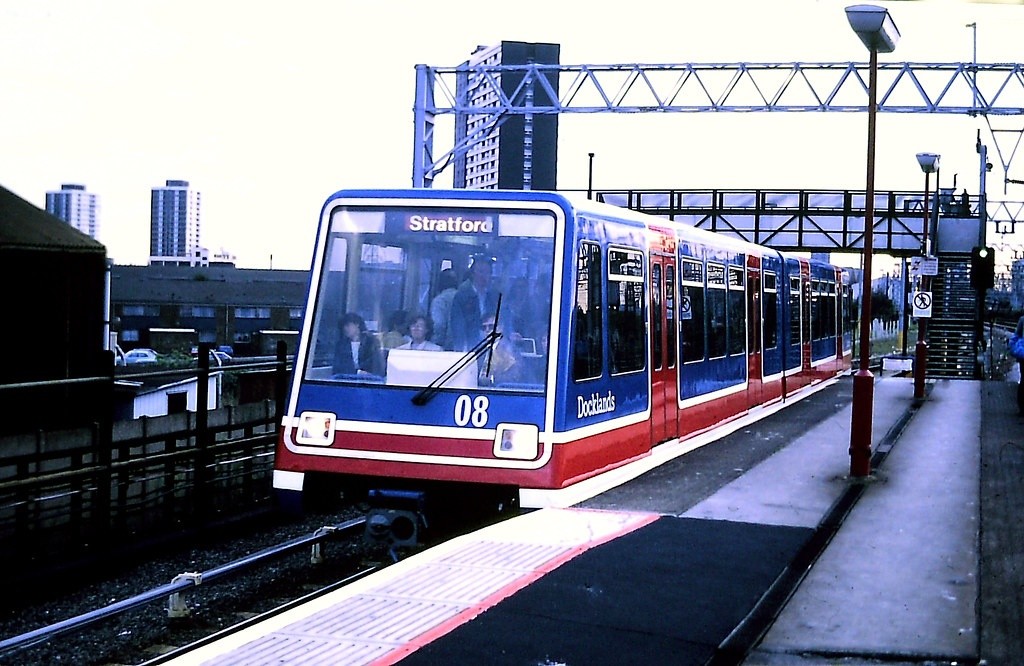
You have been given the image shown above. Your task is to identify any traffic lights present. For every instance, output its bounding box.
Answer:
[970,247,995,291]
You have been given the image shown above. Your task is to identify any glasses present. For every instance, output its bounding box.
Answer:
[480,324,498,330]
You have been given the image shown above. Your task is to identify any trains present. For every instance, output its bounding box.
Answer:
[271,185,864,568]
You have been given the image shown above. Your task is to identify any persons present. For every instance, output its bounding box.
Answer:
[388,256,552,389]
[332,311,386,380]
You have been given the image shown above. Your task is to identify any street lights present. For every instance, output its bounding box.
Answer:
[842,3,903,479]
[913,152,940,399]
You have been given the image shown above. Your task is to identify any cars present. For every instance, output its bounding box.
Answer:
[117,348,160,366]
[193,352,231,362]
